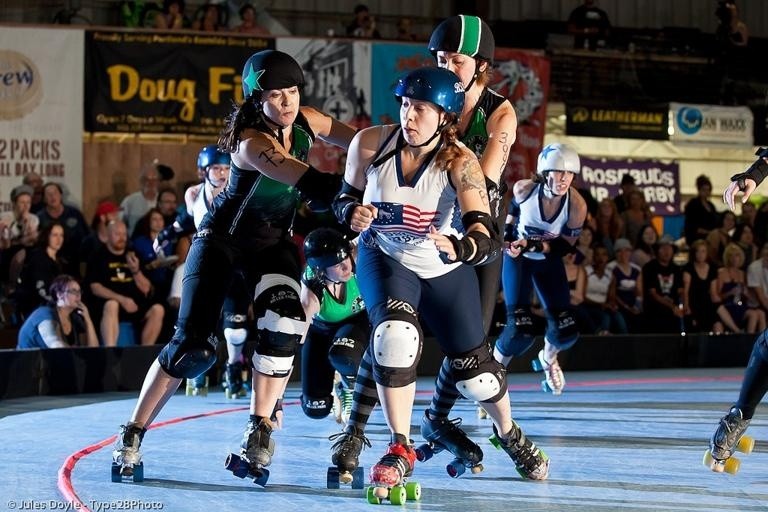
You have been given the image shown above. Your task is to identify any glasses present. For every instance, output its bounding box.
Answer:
[60,288,80,294]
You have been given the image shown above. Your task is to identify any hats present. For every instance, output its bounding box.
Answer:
[95,199,124,216]
[612,238,633,252]
[10,185,35,203]
[652,234,679,254]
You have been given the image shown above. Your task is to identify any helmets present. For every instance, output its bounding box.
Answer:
[241,49,305,130]
[426,13,496,94]
[536,143,581,197]
[393,66,465,148]
[197,144,232,189]
[302,228,357,285]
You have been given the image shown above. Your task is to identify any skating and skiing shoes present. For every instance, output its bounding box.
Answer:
[184,353,247,400]
[702,404,756,475]
[489,422,550,482]
[110,424,148,483]
[334,381,356,431]
[414,408,484,478]
[366,432,422,506]
[326,424,366,490]
[531,347,566,396]
[221,414,275,487]
